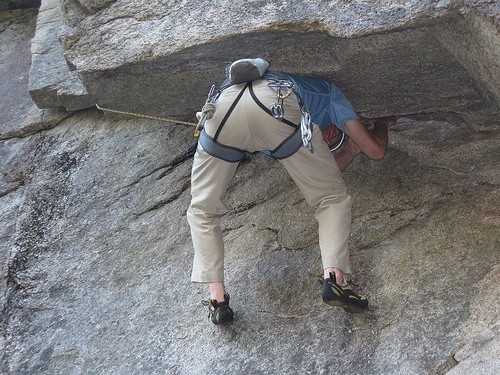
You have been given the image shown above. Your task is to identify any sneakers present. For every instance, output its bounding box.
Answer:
[317,271,369,314]
[201,294,234,324]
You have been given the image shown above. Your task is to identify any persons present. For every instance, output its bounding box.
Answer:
[186,71,397,325]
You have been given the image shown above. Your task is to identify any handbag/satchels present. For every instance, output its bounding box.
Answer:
[229,57,270,83]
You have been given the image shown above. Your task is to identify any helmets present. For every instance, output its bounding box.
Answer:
[330,133,348,154]
